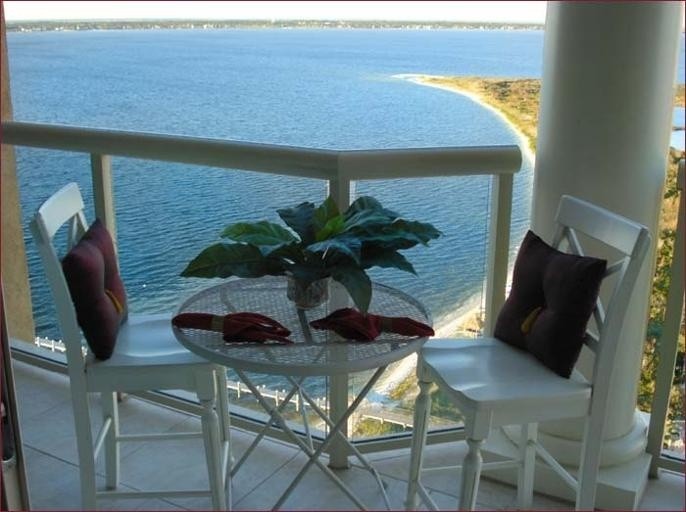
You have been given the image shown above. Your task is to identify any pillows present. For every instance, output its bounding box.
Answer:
[495,229,608,379]
[62,216,128,361]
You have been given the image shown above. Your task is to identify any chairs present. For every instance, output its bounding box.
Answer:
[408,195,651,512]
[28,182,235,511]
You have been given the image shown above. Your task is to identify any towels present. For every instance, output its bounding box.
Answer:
[171,312,297,346]
[308,307,434,344]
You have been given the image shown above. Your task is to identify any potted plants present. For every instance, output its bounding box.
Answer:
[177,191,448,320]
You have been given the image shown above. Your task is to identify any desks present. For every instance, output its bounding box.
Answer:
[171,271,434,511]
